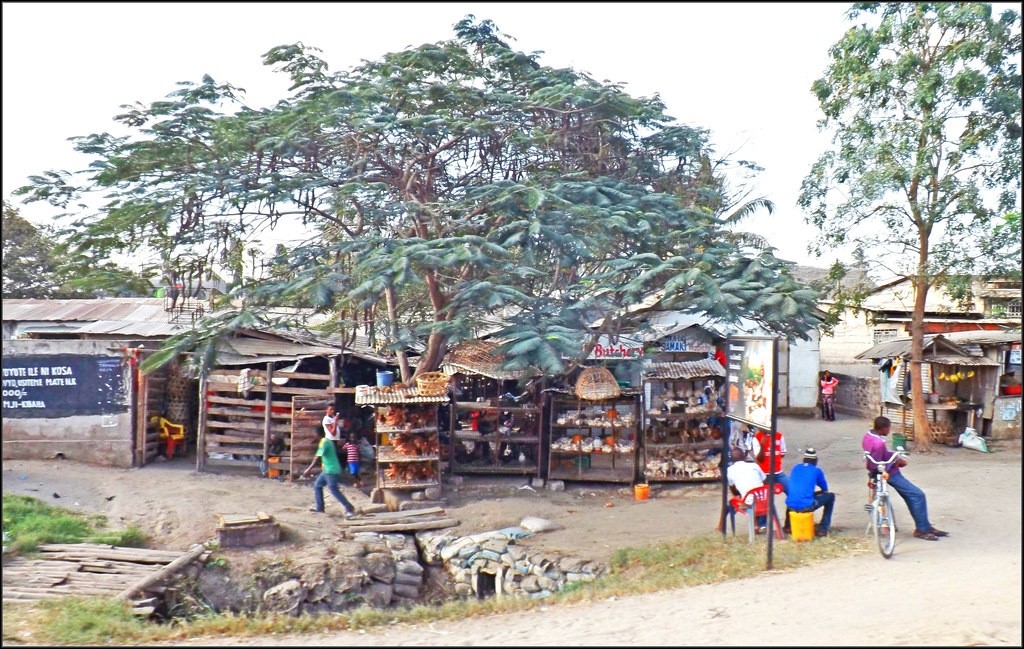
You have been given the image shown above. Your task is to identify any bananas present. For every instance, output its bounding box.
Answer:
[938,370,974,382]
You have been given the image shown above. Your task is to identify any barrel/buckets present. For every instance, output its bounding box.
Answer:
[922,391,939,403]
[789,510,816,541]
[892,432,907,452]
[573,408,618,453]
[376,371,393,387]
[634,482,650,502]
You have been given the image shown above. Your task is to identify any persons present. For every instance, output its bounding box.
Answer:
[753,429,794,532]
[342,432,365,488]
[783,448,835,535]
[715,448,767,533]
[999,367,1021,396]
[863,417,949,540]
[322,403,340,452]
[302,426,354,517]
[820,370,839,421]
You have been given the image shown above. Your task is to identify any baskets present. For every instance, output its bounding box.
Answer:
[166,355,196,443]
[415,372,451,397]
[904,421,961,446]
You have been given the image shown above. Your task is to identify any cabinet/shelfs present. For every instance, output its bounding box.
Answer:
[375,392,444,492]
[548,384,644,489]
[637,365,729,481]
[445,372,548,479]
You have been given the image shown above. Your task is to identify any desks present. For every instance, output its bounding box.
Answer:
[885,399,983,447]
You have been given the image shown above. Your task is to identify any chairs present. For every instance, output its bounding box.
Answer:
[727,485,784,539]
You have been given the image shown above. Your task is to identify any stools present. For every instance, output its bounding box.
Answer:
[789,511,814,542]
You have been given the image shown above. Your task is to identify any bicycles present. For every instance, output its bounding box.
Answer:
[863,446,910,557]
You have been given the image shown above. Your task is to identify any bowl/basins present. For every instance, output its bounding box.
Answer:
[1001,384,1022,395]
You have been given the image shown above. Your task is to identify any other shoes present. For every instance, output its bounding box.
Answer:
[761,527,766,534]
[309,508,325,513]
[929,527,948,537]
[914,529,938,541]
[816,532,827,537]
[345,510,356,518]
[782,526,791,533]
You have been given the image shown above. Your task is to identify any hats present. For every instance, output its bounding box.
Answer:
[803,448,817,460]
